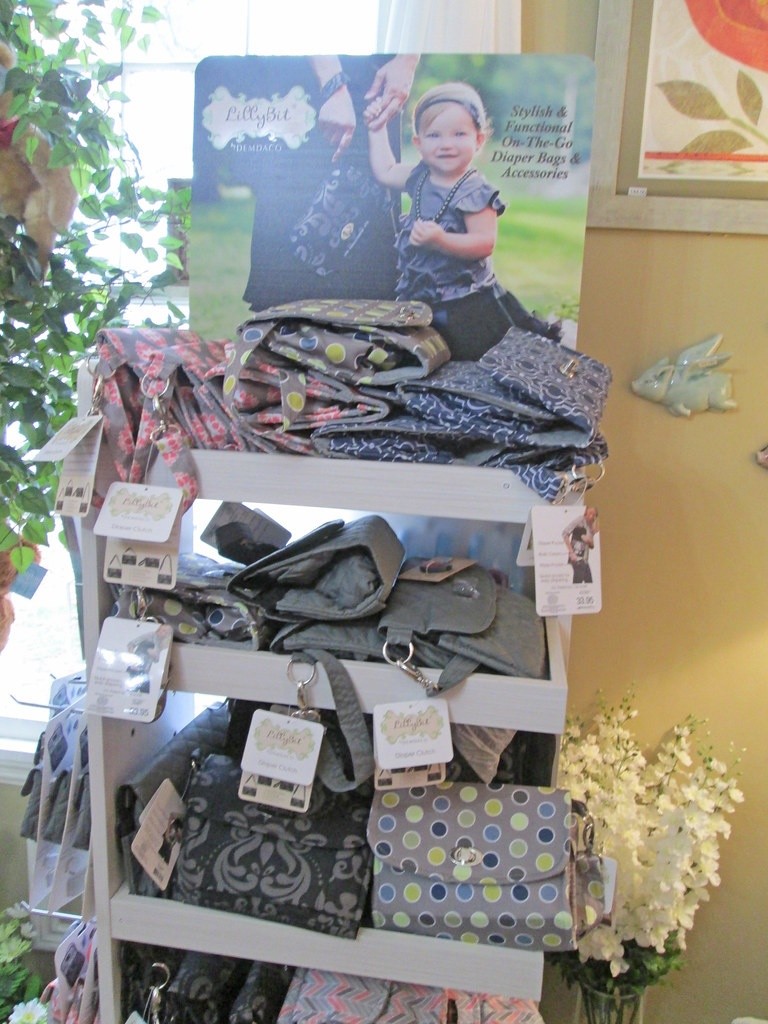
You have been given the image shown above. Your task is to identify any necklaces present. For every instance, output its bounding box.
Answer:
[417,169,477,222]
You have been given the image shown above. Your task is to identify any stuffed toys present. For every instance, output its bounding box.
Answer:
[1,42,78,279]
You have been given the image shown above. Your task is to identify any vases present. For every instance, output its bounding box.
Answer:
[574,979,647,1024]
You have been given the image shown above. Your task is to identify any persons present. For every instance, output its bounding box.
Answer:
[242,54,421,311]
[158,811,186,865]
[362,82,562,361]
[562,506,598,583]
[126,624,171,693]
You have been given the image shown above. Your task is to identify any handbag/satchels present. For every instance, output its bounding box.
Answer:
[17,300,606,1022]
[288,72,387,280]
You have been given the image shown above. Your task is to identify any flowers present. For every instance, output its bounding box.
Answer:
[554,683,745,1024]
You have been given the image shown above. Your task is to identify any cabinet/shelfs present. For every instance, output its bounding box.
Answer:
[74,361,591,1024]
